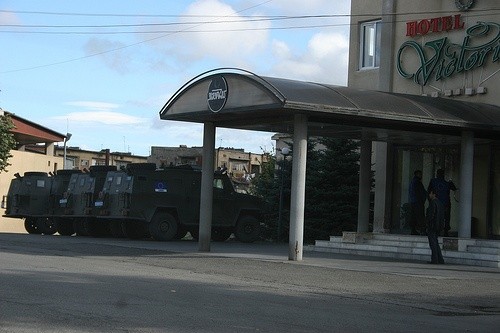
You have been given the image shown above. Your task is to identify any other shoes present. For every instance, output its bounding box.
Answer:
[420,231,428,235]
[438,258,444,264]
[427,261,438,263]
[411,231,420,235]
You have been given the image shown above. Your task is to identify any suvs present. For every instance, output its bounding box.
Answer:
[1,162,269,242]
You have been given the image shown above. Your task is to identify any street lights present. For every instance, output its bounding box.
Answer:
[276,147,289,244]
[63,133,72,170]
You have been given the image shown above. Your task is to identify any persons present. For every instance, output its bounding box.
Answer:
[426,190,445,264]
[407,169,456,235]
[215,167,227,174]
